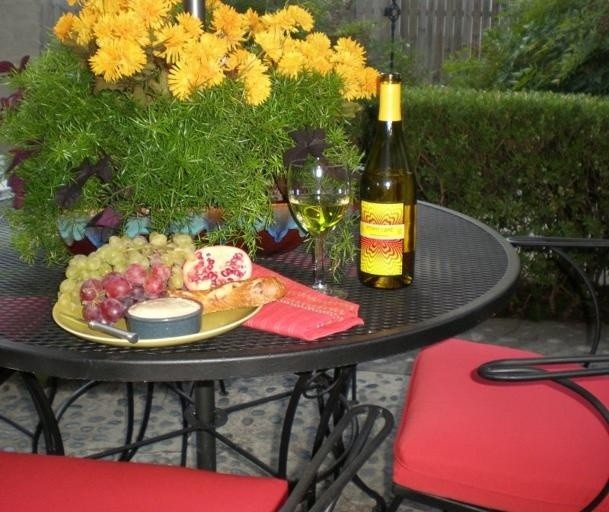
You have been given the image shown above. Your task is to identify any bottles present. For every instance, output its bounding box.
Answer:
[359,73,416,290]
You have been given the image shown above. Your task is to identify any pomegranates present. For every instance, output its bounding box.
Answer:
[182,245,253,291]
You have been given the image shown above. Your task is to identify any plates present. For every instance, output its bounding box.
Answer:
[51,295,262,348]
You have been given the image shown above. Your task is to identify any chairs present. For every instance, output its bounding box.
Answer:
[384,235,609,509]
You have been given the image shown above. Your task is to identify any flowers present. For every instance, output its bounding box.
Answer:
[5,2,386,269]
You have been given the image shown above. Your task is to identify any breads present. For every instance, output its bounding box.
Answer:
[165,277,288,315]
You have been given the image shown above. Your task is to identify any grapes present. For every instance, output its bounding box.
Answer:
[59,231,196,326]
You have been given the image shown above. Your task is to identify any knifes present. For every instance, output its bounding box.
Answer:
[58,312,138,344]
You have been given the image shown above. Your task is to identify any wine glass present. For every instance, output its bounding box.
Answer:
[287,159,352,301]
